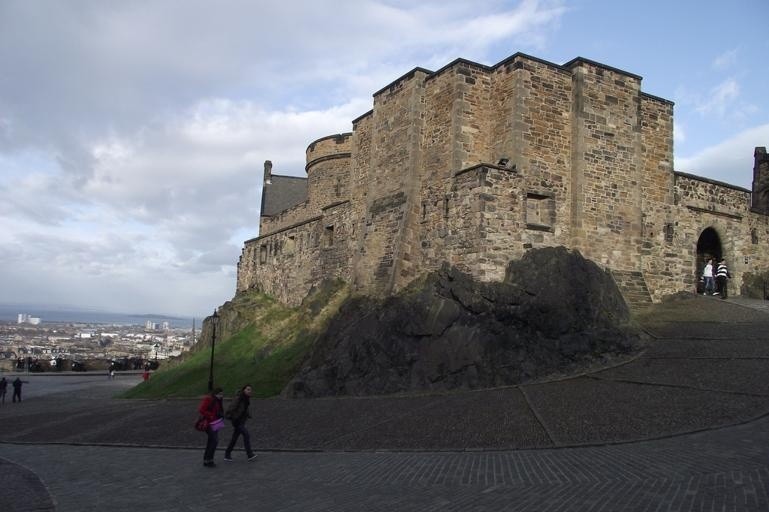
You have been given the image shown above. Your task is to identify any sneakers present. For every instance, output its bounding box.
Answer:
[248,455,258,463]
[703,292,727,299]
[203,458,233,468]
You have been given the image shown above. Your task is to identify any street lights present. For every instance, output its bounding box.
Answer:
[154,343,159,360]
[207,307,221,392]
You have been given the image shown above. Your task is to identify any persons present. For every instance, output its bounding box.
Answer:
[0,378,8,403]
[143,370,149,380]
[199,388,224,467]
[714,259,731,299]
[224,385,258,462]
[703,259,720,296]
[13,377,22,402]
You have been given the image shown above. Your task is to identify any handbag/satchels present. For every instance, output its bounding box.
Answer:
[225,401,233,419]
[195,417,209,431]
[210,418,224,432]
